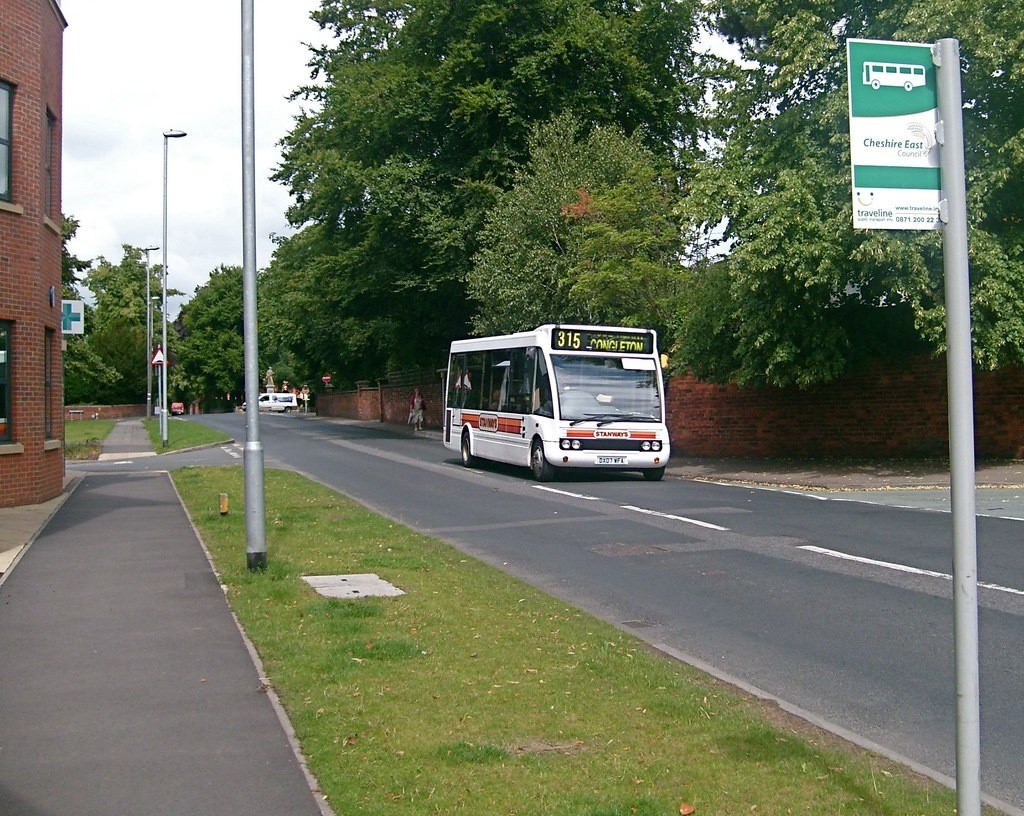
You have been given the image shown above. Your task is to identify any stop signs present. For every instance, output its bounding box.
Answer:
[322,372,331,383]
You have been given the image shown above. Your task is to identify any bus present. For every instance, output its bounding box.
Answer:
[443,324,670,482]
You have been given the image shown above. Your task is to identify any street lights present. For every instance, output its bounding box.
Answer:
[150,296,160,357]
[145,246,160,418]
[163,129,187,448]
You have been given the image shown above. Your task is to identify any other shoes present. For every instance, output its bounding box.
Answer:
[415,428,418,431]
[419,428,423,430]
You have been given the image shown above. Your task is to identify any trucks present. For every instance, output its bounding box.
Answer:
[242,393,298,413]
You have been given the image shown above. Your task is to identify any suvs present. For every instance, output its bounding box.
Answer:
[171,403,184,415]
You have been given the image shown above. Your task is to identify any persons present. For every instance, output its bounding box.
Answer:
[410,388,426,430]
[266,367,274,385]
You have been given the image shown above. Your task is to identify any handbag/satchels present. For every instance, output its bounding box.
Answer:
[407,412,413,424]
